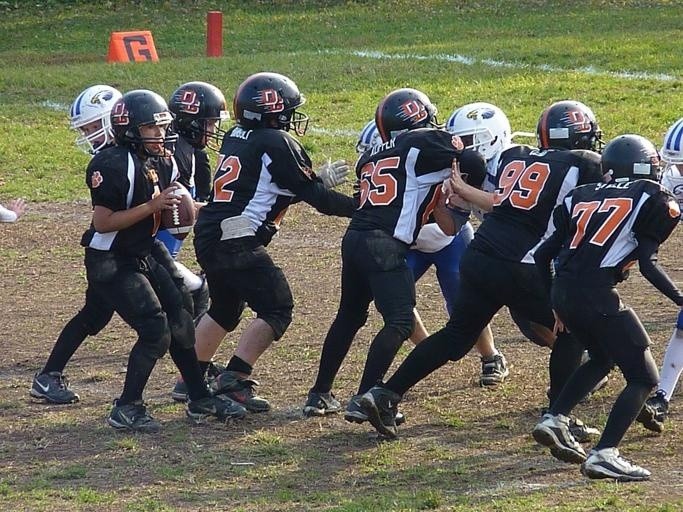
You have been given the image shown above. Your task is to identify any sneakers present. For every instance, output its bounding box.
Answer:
[171,360,270,420]
[344,383,405,438]
[29,371,79,404]
[580,445,653,482]
[479,351,509,388]
[107,398,159,432]
[532,412,601,464]
[636,393,668,433]
[303,389,343,417]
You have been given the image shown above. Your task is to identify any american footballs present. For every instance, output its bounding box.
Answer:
[162,181,195,239]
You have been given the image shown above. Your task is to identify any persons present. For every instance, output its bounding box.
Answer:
[28,72,356,430]
[302,86,682,435]
[0,194,27,225]
[533,133,682,482]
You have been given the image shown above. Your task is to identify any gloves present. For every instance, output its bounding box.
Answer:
[321,157,349,187]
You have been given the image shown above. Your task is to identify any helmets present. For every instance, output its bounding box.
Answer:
[537,100,602,153]
[356,119,382,153]
[445,102,511,160]
[658,118,683,219]
[601,133,661,182]
[110,90,179,159]
[168,81,229,152]
[233,72,309,137]
[67,84,122,156]
[375,88,439,143]
